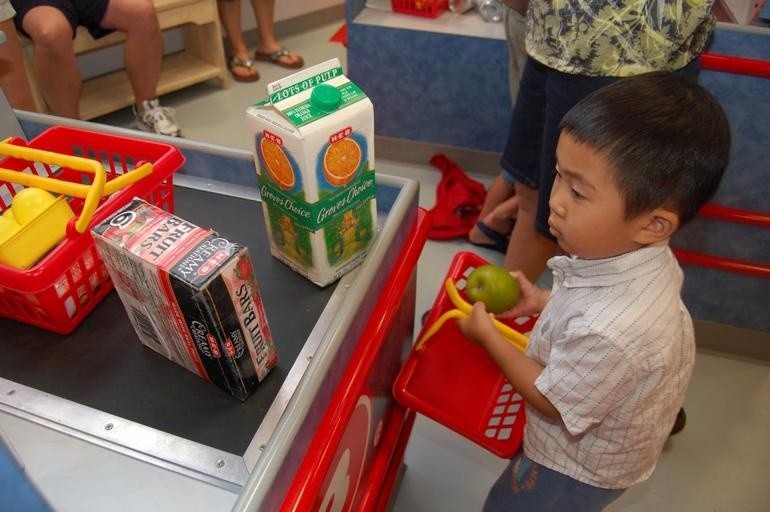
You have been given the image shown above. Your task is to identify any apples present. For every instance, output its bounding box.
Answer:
[467,264,519,313]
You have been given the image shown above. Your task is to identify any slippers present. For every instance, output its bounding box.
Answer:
[228,56,259,81]
[255,47,303,68]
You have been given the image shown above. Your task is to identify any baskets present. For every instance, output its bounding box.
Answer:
[0,127,185,333]
[391,0,448,17]
[392,252,542,457]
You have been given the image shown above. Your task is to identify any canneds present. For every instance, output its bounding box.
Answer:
[448,0,475,13]
[476,0,502,23]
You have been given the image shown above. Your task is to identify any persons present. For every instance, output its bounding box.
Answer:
[466,1,530,255]
[501,1,719,436]
[216,0,305,82]
[454,72,732,511]
[9,0,183,139]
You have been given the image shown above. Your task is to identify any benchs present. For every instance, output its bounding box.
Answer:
[17,0,229,122]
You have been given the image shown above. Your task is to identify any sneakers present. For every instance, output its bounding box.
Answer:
[133,99,179,136]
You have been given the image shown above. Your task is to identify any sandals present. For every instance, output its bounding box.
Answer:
[467,220,512,254]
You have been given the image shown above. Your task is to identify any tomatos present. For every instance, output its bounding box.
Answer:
[233,258,251,280]
